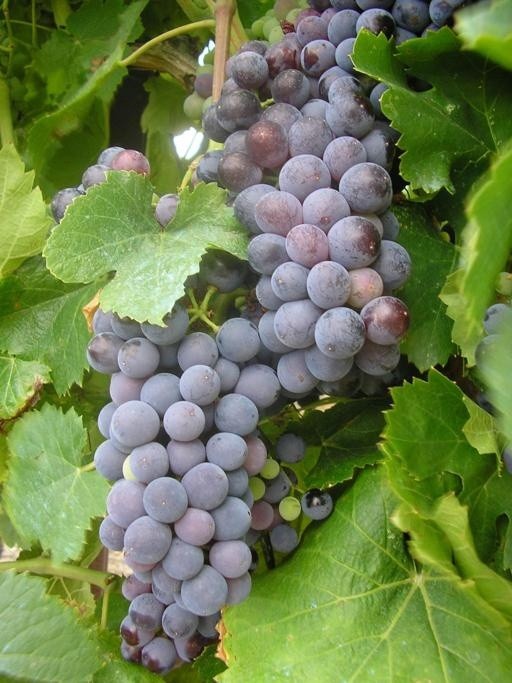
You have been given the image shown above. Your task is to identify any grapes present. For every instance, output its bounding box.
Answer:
[52,0,512,669]
